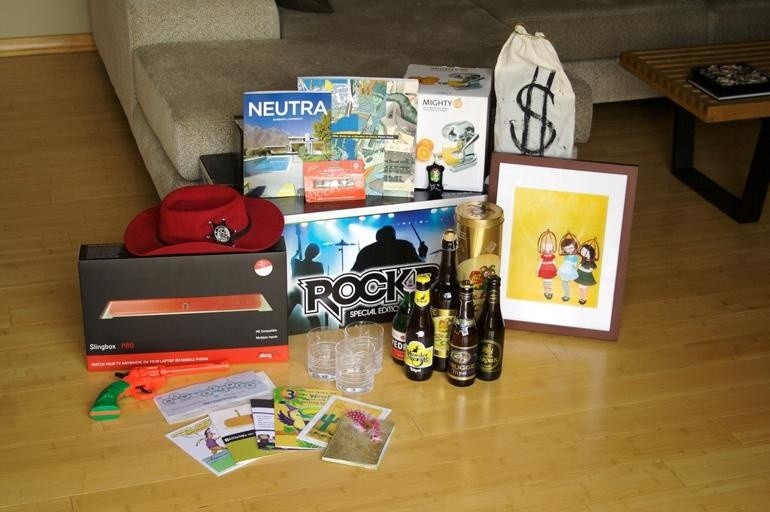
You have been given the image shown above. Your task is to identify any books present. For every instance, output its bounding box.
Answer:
[324,411,396,471]
[243,91,333,198]
[250,399,276,448]
[208,402,296,465]
[296,394,391,448]
[274,386,343,450]
[165,416,251,477]
[297,76,419,197]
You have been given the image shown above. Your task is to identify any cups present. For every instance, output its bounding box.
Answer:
[335,339,374,396]
[305,326,345,381]
[345,321,384,375]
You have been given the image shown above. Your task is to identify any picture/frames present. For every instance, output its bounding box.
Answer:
[489,152,640,342]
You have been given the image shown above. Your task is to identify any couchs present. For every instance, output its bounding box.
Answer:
[86,0,768,203]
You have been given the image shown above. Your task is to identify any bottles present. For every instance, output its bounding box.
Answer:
[478,275,505,381]
[447,280,478,387]
[404,274,434,381]
[455,201,504,319]
[430,230,458,372]
[391,284,415,365]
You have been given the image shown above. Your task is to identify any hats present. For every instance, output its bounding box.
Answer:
[124,184,285,256]
